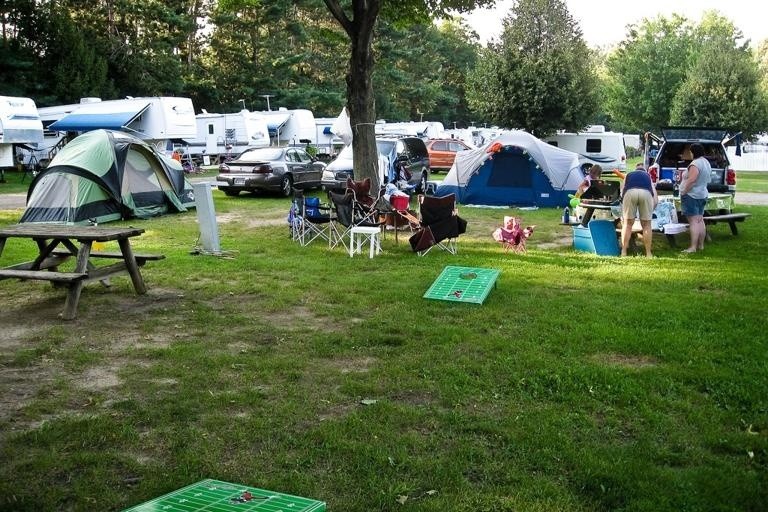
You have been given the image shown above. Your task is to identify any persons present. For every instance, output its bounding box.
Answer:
[620,166,658,259]
[678,143,712,254]
[172,150,181,162]
[577,164,602,194]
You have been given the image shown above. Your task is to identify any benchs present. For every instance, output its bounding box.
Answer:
[703,213,752,219]
[51,248,166,261]
[0,269,85,282]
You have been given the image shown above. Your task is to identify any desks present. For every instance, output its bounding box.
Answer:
[0,222,146,321]
[579,192,738,248]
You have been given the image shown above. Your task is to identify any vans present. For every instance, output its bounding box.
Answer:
[321,136,431,194]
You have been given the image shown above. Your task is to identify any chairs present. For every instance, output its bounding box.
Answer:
[292,178,467,258]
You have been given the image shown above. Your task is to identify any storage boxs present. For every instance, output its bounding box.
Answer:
[572,219,620,258]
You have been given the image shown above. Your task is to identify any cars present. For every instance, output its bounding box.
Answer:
[421,138,477,173]
[216,145,327,194]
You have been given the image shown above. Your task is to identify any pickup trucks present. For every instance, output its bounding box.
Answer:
[649,126,736,202]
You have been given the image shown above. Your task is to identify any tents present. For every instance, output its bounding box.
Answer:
[16,127,196,227]
[432,130,587,207]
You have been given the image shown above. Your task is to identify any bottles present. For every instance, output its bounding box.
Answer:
[673,181,679,197]
[564,208,569,223]
[673,161,681,181]
